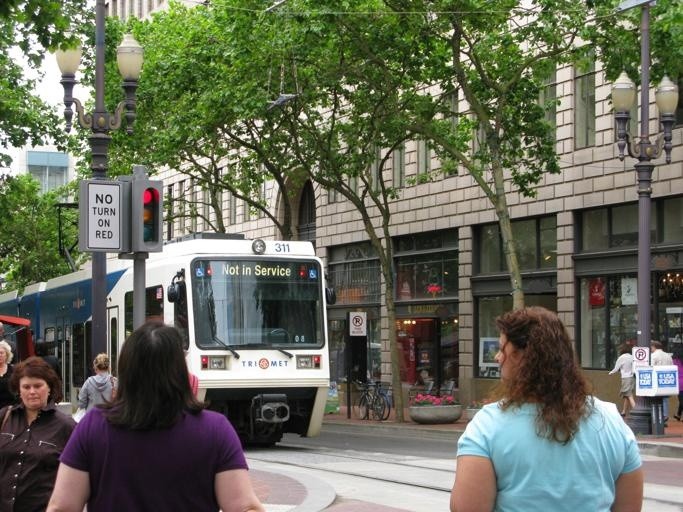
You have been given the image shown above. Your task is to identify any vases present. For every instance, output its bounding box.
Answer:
[408,404,480,424]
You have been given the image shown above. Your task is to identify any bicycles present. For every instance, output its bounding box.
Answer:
[351,379,391,421]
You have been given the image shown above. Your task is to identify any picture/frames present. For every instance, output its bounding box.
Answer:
[478,337,502,367]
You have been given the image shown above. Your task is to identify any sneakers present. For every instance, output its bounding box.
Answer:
[663,413,683,427]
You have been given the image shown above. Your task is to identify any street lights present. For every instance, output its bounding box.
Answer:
[56,0,144,377]
[610,0,679,436]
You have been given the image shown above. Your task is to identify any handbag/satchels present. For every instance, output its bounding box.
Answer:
[110,377,117,401]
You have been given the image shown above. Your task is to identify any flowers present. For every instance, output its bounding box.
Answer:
[423,282,447,298]
[468,399,493,409]
[407,393,458,405]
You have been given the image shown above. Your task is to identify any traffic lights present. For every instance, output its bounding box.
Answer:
[132,179,164,252]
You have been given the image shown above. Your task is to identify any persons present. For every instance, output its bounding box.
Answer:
[0,356,78,512]
[0,340,21,409]
[448,305,645,511]
[650,340,674,426]
[671,346,682,422]
[42,318,265,512]
[71,352,117,423]
[608,344,635,415]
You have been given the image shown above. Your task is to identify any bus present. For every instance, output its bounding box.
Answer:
[0,232,336,448]
